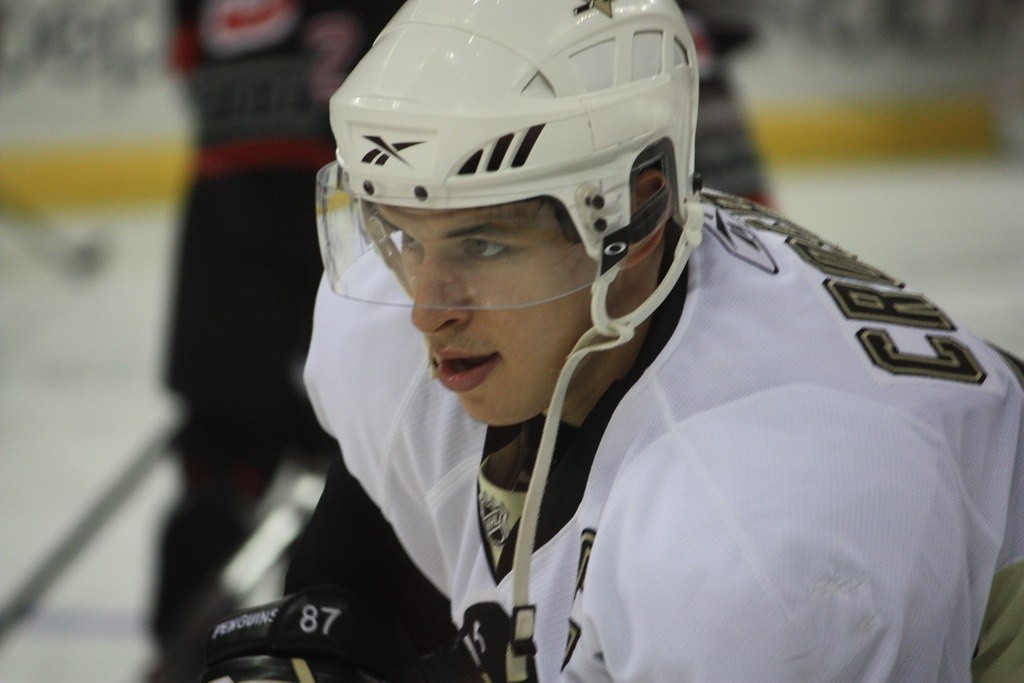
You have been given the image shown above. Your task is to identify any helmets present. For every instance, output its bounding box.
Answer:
[316,0,700,310]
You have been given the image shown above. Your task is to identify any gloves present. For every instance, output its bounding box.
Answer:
[204,586,422,683]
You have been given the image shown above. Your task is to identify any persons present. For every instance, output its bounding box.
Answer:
[265,0,1021,683]
[142,0,406,659]
